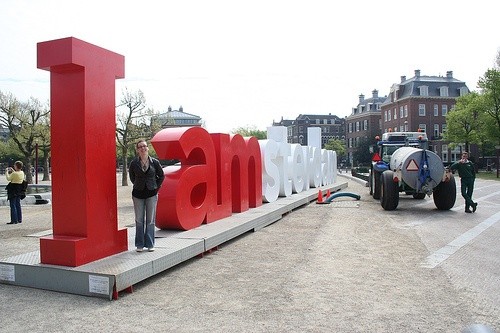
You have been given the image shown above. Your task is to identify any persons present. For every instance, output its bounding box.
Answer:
[129,140,165,252]
[31,166,35,176]
[5,161,24,224]
[449,152,477,213]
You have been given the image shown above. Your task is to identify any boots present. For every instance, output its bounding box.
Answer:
[471,203,477,212]
[465,207,471,213]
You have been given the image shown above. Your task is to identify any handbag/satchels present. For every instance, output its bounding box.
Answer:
[20,180,28,191]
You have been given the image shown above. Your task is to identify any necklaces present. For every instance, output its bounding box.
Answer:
[141,158,149,172]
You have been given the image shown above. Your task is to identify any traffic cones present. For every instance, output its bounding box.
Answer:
[326,189,334,202]
[316,190,324,204]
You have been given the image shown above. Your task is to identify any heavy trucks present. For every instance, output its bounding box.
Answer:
[369,131,458,211]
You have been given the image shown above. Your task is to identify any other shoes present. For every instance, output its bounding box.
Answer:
[136,247,143,251]
[7,220,21,224]
[148,248,155,252]
